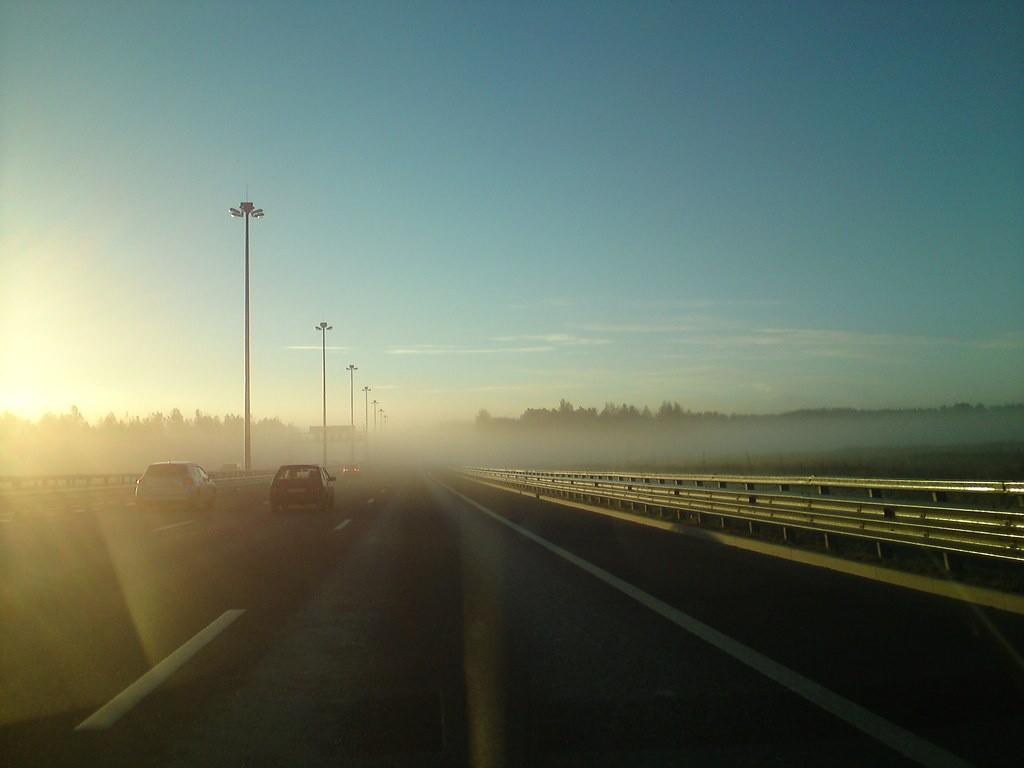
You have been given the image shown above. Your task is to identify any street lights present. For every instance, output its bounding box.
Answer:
[314,323,334,468]
[362,387,389,450]
[345,364,357,466]
[229,202,264,469]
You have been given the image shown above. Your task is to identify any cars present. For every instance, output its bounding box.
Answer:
[222,462,243,474]
[269,464,338,512]
[135,461,219,511]
[343,465,361,476]
